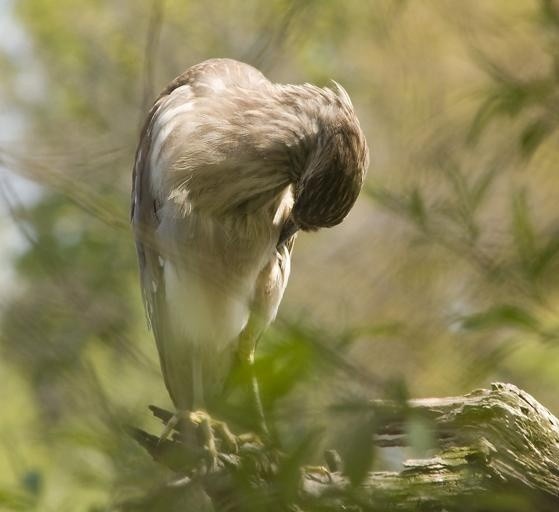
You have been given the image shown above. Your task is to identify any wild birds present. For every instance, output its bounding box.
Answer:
[131,58,369,473]
